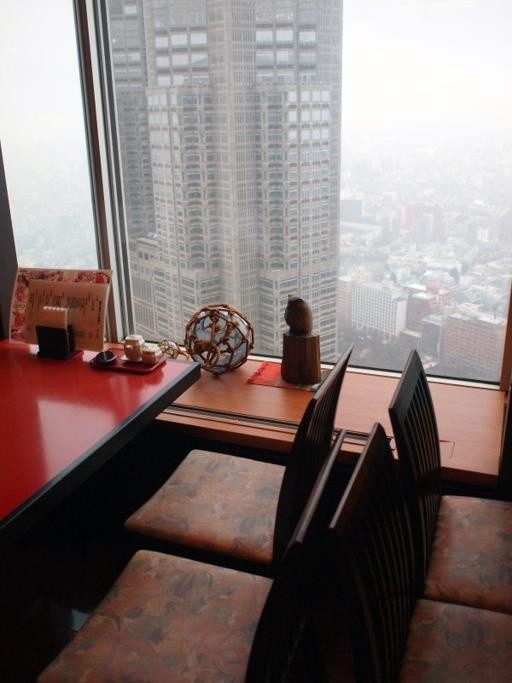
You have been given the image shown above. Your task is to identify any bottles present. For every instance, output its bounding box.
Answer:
[140,343,162,365]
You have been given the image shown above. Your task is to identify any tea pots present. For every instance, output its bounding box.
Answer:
[123,333,148,362]
